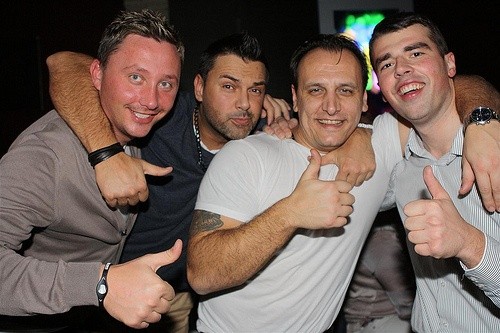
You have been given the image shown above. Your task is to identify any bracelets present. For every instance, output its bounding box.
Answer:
[96,263,113,310]
[87,142,123,168]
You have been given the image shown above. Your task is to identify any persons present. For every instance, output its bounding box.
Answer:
[0,7,291,333]
[187,33,500,333]
[46,18,376,333]
[262,14,500,333]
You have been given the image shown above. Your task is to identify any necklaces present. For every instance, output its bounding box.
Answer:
[193,109,209,170]
[291,133,295,140]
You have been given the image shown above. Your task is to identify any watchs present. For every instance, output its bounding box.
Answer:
[460,106,499,127]
